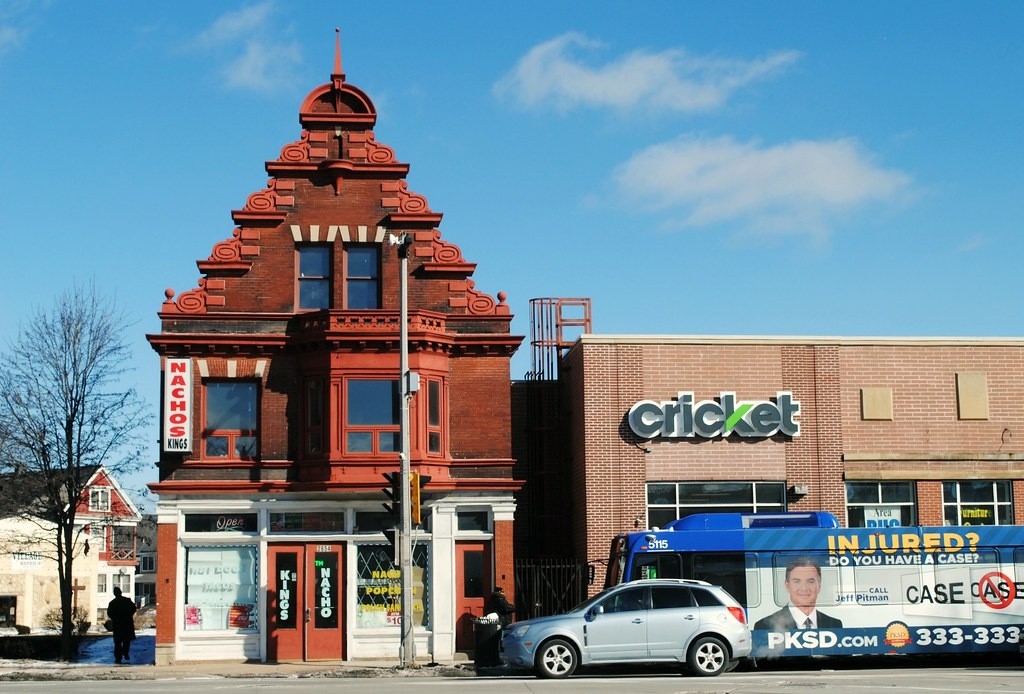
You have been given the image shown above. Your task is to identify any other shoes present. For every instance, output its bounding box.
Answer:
[123,649,130,660]
[115,659,121,663]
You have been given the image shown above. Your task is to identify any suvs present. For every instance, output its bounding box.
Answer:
[499,577,751,680]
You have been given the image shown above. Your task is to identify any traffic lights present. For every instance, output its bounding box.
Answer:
[410,472,432,526]
[381,470,402,523]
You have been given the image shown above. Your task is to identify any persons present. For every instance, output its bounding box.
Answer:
[483,587,517,653]
[754,557,843,631]
[107,586,137,664]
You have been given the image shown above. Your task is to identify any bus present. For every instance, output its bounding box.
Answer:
[584,513,1024,668]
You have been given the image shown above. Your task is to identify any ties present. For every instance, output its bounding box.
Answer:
[803,618,813,629]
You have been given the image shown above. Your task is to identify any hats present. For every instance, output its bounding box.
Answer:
[114,587,122,594]
[496,588,504,593]
